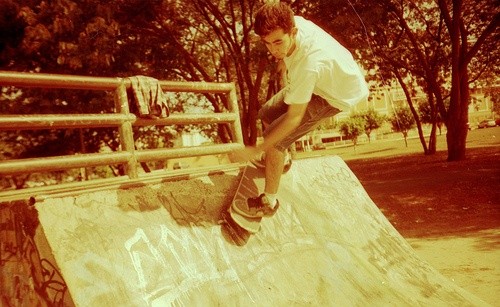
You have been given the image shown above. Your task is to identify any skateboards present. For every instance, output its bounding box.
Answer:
[221,147,277,233]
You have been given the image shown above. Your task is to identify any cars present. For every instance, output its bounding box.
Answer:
[478,119,495,129]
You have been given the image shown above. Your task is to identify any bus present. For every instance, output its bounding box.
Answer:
[295,134,354,151]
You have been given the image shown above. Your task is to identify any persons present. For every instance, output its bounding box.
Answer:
[232,1,368,220]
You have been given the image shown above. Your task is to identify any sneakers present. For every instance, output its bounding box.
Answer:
[232,193,279,218]
[249,151,291,170]
